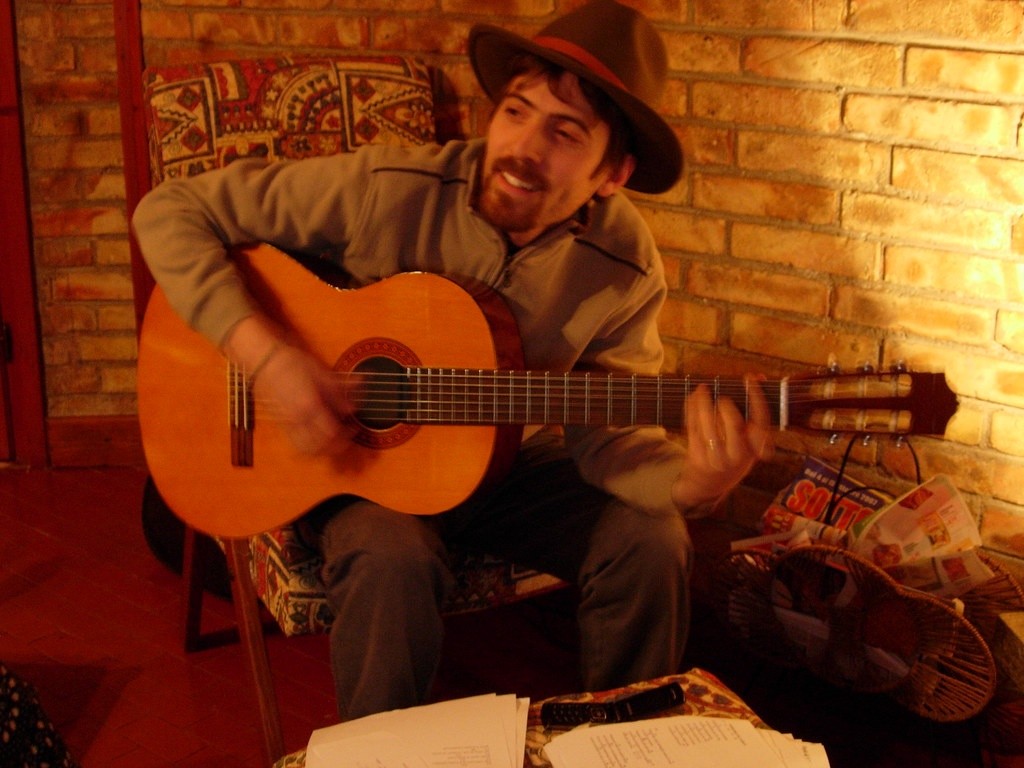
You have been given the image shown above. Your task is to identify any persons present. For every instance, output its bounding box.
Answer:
[131,0,777,724]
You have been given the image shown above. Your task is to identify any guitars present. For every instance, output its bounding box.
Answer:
[134,239,962,540]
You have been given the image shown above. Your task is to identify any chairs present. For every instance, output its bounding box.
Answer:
[141,50,567,762]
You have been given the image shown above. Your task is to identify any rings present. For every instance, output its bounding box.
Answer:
[704,439,721,450]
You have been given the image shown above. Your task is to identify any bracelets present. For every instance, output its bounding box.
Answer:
[245,337,283,382]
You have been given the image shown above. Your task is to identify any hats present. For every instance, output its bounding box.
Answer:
[467,5,684,194]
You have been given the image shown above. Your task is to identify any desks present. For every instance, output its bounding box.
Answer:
[273,667,770,768]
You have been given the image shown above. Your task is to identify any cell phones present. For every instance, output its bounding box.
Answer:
[541,682,685,729]
